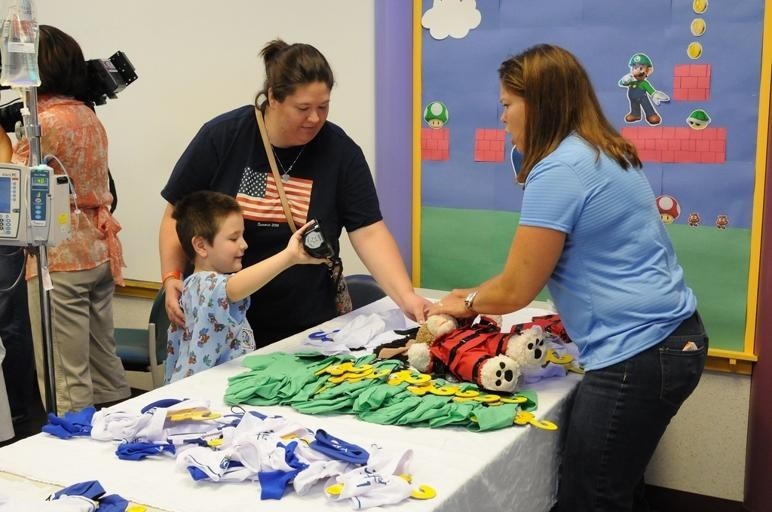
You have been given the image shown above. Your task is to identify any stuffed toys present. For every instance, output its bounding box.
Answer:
[398,311,551,376]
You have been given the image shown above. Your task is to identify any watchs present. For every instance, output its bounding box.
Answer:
[464,289,482,312]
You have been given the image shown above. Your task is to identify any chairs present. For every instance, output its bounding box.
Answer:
[112,283,168,397]
[342,274,386,312]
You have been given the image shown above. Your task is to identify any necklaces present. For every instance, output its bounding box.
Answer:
[261,114,307,181]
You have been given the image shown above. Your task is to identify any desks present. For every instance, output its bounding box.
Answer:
[1,283,582,509]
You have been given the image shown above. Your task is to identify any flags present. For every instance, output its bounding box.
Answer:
[235,165,315,228]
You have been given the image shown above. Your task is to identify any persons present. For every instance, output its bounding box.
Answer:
[148,38,434,359]
[425,43,711,509]
[1,23,133,420]
[163,191,332,384]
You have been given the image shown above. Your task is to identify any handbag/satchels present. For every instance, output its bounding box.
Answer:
[329,258,353,315]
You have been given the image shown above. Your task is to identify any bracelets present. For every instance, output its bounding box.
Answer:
[165,270,184,285]
